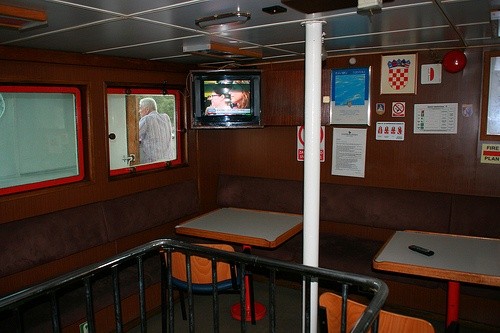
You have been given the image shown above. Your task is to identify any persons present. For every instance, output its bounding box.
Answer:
[205,80,250,116]
[139,98,173,164]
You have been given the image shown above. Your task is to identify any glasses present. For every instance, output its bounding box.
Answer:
[208,94,218,98]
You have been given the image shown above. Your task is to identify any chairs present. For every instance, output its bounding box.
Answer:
[317,292,435,333]
[159,242,257,333]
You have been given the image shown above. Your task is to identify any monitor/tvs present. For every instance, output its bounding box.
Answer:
[191,71,262,126]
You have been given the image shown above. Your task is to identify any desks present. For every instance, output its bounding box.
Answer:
[372,228,500,328]
[175,206,304,322]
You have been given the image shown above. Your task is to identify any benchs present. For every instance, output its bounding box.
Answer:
[0,175,201,333]
[215,174,500,323]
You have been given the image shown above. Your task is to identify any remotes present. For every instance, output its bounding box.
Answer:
[409,245,435,255]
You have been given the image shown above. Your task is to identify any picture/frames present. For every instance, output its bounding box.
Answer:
[380,53,418,95]
